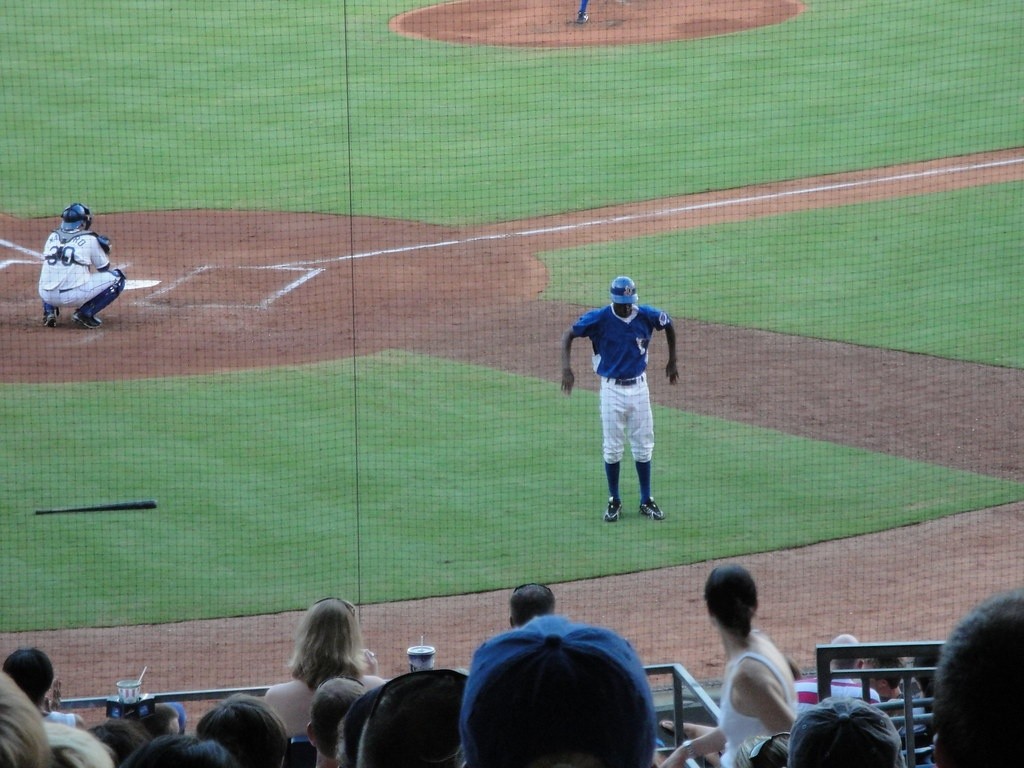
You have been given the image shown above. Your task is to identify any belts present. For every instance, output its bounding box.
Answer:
[606,376,643,386]
[59,287,72,293]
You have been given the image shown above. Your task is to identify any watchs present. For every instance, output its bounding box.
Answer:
[683,740,698,758]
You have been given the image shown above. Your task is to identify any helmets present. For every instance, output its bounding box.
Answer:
[62,205,85,230]
[610,276,638,304]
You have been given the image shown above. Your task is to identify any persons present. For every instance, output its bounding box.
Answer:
[0,649,286,768]
[658,634,936,768]
[658,565,798,768]
[39,204,125,328]
[266,598,386,738]
[510,583,554,628]
[306,669,467,768]
[576,0,589,24]
[561,277,680,522]
[458,615,655,768]
[932,586,1024,768]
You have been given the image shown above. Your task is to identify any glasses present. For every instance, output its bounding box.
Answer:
[369,668,468,717]
[514,582,553,597]
[749,731,791,768]
[314,596,356,614]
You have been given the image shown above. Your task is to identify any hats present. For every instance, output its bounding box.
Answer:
[787,697,908,768]
[459,614,656,768]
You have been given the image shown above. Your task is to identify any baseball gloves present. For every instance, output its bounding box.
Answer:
[100,234,112,256]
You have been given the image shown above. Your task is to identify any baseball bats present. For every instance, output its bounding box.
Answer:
[35,499,158,515]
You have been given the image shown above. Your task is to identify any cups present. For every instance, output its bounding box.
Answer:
[406,645,437,673]
[115,679,142,704]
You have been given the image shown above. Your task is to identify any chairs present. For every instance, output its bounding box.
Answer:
[898,723,934,765]
[281,735,317,768]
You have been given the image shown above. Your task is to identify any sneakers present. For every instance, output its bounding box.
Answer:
[71,310,102,329]
[43,314,57,327]
[640,497,665,520]
[576,11,589,23]
[603,497,622,522]
